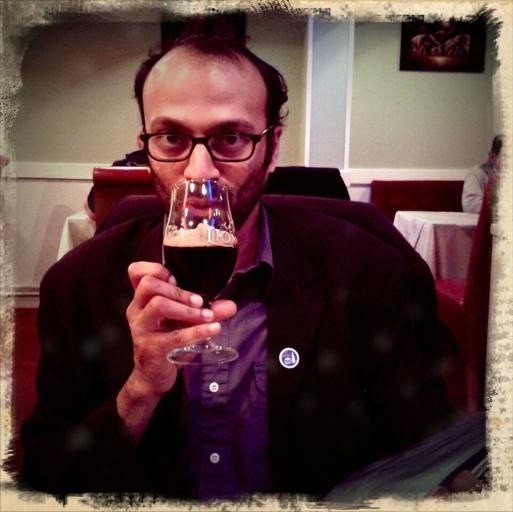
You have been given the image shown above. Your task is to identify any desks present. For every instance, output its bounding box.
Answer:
[57,211,97,259]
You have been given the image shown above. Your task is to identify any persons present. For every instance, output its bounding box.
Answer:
[16,28,455,499]
[460,135,503,213]
[84,130,153,216]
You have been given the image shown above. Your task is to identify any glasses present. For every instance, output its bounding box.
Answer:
[140,125,274,162]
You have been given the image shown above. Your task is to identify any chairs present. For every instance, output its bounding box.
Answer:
[92,194,437,306]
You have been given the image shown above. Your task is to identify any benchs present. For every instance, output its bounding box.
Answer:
[370,178,496,410]
[92,165,351,234]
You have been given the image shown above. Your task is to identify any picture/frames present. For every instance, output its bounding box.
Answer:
[398,22,487,72]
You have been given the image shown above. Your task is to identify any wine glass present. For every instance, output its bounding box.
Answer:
[159,178,241,368]
